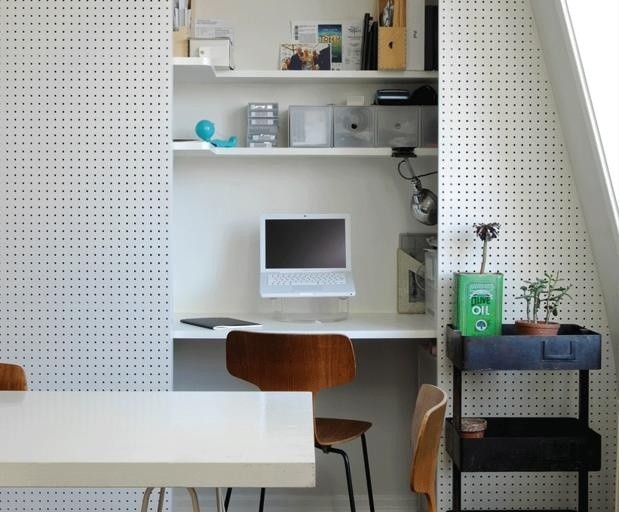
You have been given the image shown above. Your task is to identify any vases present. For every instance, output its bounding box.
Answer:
[450,418,488,438]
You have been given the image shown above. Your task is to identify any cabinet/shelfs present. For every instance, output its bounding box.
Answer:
[171,56,437,157]
[445,323,602,512]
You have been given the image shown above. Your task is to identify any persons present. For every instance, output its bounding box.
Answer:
[287,47,303,69]
[311,50,318,69]
[281,57,291,70]
[298,48,313,69]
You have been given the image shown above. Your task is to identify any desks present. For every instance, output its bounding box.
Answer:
[0,391,314,512]
[173,314,436,340]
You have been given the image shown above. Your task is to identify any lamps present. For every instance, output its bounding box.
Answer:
[389,145,437,227]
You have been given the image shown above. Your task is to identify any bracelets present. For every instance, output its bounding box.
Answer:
[298,55,301,57]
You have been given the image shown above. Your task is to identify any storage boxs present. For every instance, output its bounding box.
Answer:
[189,39,234,71]
[286,102,438,148]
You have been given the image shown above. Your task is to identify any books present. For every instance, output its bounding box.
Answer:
[359,12,370,68]
[367,31,371,69]
[171,137,211,149]
[172,0,192,32]
[179,316,263,330]
[370,20,377,70]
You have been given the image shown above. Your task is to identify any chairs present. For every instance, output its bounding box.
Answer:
[0,362,28,391]
[409,384,448,512]
[223,329,375,512]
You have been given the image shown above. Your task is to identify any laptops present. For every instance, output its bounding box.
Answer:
[259,212,355,299]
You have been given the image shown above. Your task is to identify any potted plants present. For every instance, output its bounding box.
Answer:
[453,222,504,337]
[514,269,573,336]
